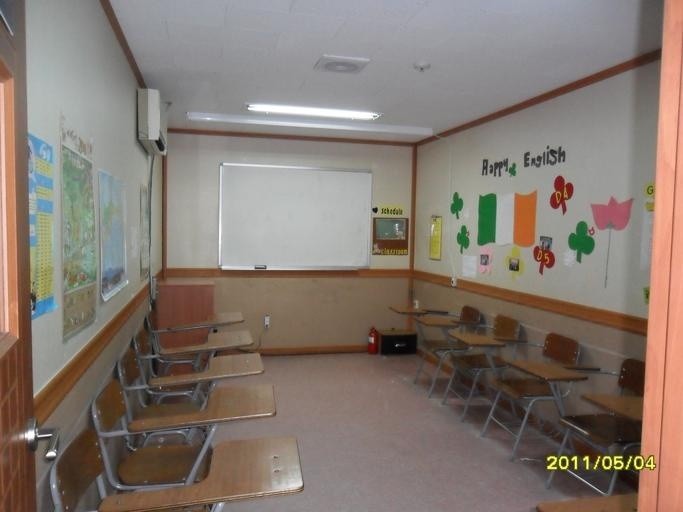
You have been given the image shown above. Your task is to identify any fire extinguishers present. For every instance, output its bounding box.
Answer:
[368,327,379,354]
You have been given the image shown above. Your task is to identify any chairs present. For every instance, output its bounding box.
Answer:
[476,331,580,462]
[439,313,521,423]
[540,358,644,496]
[414,305,484,401]
[48,307,211,512]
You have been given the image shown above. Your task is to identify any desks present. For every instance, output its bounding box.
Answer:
[146,350,266,389]
[97,432,303,511]
[581,393,645,424]
[168,309,246,338]
[504,357,605,386]
[157,329,254,358]
[389,305,449,343]
[450,328,509,356]
[415,314,455,330]
[126,379,278,442]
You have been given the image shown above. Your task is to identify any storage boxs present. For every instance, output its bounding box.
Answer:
[378,329,417,355]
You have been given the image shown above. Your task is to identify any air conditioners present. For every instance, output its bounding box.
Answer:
[136,86,168,156]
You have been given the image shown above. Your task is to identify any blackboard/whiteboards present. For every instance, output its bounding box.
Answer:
[217,162,374,271]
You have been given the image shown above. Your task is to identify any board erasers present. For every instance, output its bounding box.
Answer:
[254,265,267,270]
